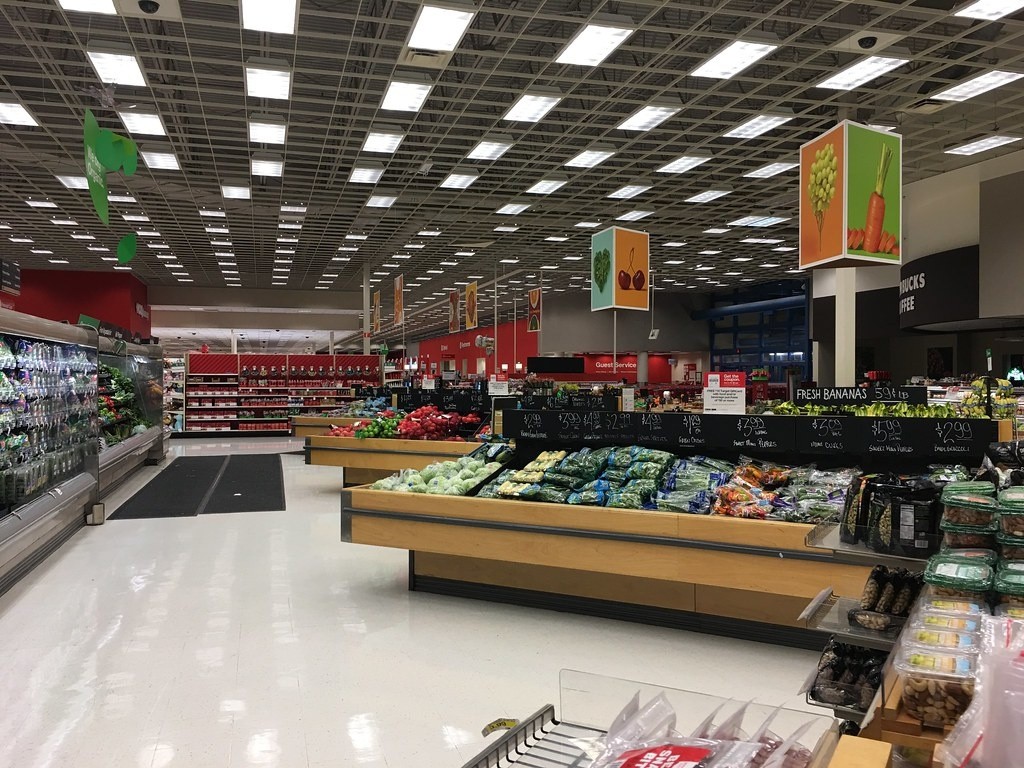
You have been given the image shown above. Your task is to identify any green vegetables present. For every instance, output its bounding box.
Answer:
[538,445,731,514]
[98,361,153,447]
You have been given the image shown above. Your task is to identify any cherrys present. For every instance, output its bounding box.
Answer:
[619,270,631,289]
[633,270,645,290]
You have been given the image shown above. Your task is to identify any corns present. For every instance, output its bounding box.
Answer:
[497,450,566,495]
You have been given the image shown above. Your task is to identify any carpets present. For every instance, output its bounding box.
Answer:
[199,453,286,514]
[106,455,228,519]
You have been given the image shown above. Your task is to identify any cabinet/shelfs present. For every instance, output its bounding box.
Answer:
[0,307,1024,768]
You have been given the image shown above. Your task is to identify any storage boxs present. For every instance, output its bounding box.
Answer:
[994,570,1024,608]
[940,548,998,569]
[902,626,981,654]
[893,645,978,724]
[996,559,1024,572]
[920,594,980,614]
[939,513,999,548]
[940,492,1000,524]
[998,489,1024,506]
[923,554,995,607]
[997,505,1024,537]
[908,608,982,635]
[943,481,996,497]
[996,531,1024,560]
[994,603,1024,620]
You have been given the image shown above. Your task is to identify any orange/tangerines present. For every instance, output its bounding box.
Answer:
[359,413,402,438]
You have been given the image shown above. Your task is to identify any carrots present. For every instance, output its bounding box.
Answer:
[862,191,885,252]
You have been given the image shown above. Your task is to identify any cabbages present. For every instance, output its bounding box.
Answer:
[368,457,501,496]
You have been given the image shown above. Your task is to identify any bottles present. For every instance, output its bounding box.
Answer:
[239,366,379,386]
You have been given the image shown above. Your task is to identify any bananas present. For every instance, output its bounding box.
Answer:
[775,376,1024,430]
